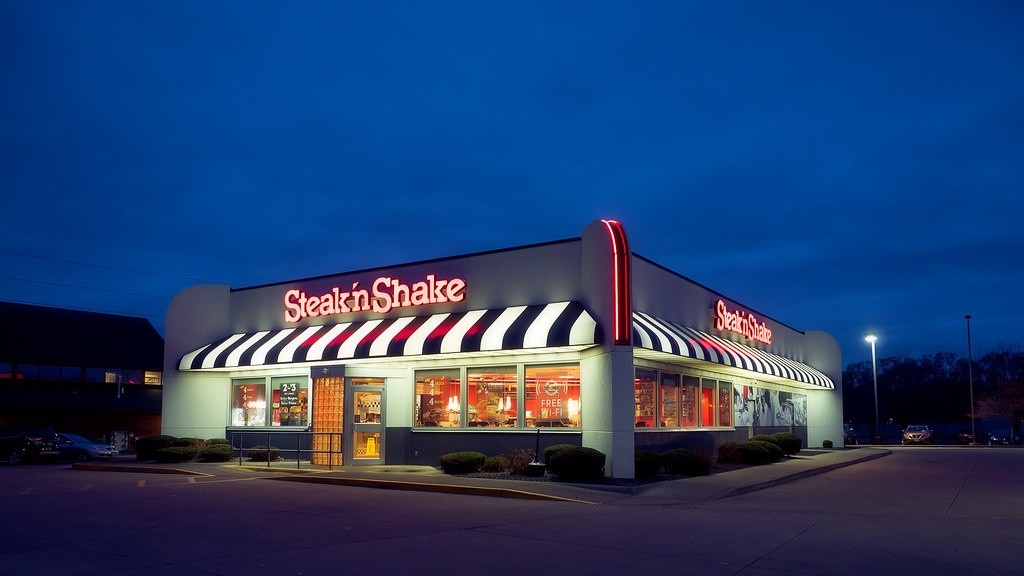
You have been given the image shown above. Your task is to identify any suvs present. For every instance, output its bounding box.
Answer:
[0,425,63,465]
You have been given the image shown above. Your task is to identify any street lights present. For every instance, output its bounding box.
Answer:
[964,314,977,443]
[864,332,883,444]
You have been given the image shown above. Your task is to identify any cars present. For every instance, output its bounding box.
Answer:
[960,428,983,443]
[989,431,1010,445]
[57,433,120,463]
[901,424,935,446]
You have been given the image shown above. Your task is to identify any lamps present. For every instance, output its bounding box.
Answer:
[448,379,458,410]
[498,377,511,411]
